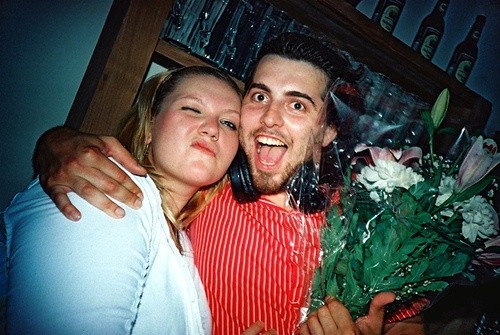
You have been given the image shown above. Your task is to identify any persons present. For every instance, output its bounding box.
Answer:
[32,32,432,335]
[0,65,276,335]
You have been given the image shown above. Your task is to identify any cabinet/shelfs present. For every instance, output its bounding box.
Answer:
[65,0,493,154]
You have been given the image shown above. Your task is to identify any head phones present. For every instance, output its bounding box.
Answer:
[228,143,337,214]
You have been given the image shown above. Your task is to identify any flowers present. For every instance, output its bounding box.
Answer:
[294,87,499,323]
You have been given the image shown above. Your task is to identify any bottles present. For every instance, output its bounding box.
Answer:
[445,15,487,85]
[341,80,425,152]
[371,0,406,34]
[409,0,450,62]
